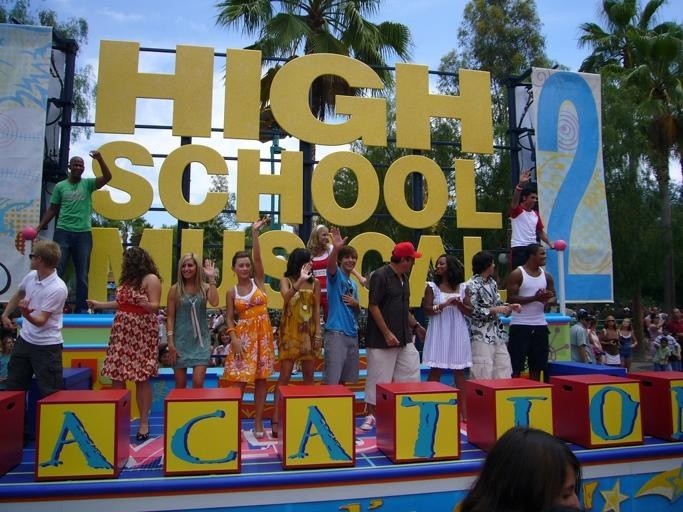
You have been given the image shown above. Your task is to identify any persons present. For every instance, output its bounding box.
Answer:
[463,252,522,380]
[509,245,557,382]
[570,298,682,371]
[455,425,582,511]
[87,246,161,442]
[350,243,426,432]
[512,166,555,269]
[36,151,111,312]
[163,254,218,389]
[271,225,362,438]
[420,255,474,422]
[0,218,67,442]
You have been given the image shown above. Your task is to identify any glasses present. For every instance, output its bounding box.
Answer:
[29,254,36,259]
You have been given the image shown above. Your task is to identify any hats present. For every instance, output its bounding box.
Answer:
[392,242,422,259]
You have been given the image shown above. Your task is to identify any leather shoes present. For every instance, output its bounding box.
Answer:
[270,419,278,438]
[255,431,264,439]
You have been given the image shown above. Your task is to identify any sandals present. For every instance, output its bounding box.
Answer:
[137,432,150,440]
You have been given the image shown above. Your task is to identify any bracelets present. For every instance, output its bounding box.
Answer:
[515,185,523,192]
[226,328,236,334]
[209,281,217,285]
[432,305,443,313]
[166,331,173,336]
